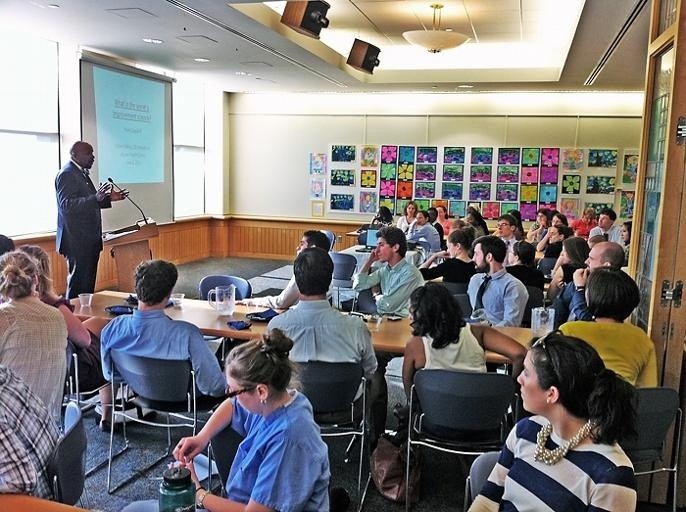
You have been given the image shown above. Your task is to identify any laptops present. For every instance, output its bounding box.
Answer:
[356,227,379,254]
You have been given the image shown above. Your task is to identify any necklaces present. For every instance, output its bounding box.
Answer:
[535,415,602,466]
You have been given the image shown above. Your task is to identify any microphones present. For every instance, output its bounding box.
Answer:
[108,177,149,224]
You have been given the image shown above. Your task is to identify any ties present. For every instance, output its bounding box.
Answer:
[84,172,97,194]
[474,276,492,311]
[503,241,509,266]
[567,309,576,323]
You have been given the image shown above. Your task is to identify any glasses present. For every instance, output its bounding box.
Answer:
[537,215,547,218]
[496,223,511,227]
[226,385,258,399]
[532,329,565,391]
[552,220,563,223]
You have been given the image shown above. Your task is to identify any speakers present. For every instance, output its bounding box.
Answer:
[345,38,380,75]
[278,1,331,40]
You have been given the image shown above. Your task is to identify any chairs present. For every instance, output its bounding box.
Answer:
[50,401,88,505]
[105,349,217,494]
[447,283,478,322]
[464,451,504,512]
[206,427,244,497]
[619,387,682,511]
[198,276,252,359]
[328,254,356,287]
[405,368,514,511]
[290,362,373,512]
[356,268,383,314]
[319,230,337,253]
[521,285,545,327]
[64,340,128,476]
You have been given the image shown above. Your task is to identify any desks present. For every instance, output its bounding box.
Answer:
[68,289,534,362]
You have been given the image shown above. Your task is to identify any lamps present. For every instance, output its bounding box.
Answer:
[402,5,470,54]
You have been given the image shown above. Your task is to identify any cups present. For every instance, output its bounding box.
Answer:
[170,293,185,311]
[470,309,488,326]
[246,300,258,313]
[530,306,556,338]
[79,294,92,307]
[367,318,378,332]
[207,284,236,316]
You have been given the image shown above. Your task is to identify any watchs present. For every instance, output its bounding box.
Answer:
[196,489,211,508]
[574,285,584,291]
[371,290,381,299]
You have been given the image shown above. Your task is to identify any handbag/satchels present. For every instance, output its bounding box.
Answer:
[369,434,421,503]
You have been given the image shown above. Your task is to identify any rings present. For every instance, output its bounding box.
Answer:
[177,442,182,449]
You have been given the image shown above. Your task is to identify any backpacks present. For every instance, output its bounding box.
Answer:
[369,428,422,507]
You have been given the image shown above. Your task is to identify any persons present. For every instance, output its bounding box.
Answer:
[505,240,545,302]
[619,221,632,269]
[55,141,130,300]
[264,246,388,454]
[525,208,556,244]
[545,236,591,303]
[585,208,621,248]
[0,250,68,438]
[465,237,528,329]
[541,225,574,258]
[356,206,396,234]
[396,200,418,239]
[427,207,444,252]
[99,259,229,401]
[386,283,528,479]
[172,328,388,511]
[462,207,488,242]
[586,235,607,251]
[567,208,599,239]
[0,363,67,499]
[0,233,14,257]
[449,220,475,261]
[236,231,343,310]
[505,209,527,242]
[558,268,658,392]
[351,229,424,371]
[435,206,455,241]
[537,213,569,253]
[406,210,441,269]
[18,244,113,435]
[497,213,519,269]
[418,226,477,284]
[550,241,626,330]
[465,332,639,511]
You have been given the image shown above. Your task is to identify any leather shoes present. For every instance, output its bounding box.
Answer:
[99,421,123,434]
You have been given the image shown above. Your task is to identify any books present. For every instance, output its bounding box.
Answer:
[631,95,668,325]
